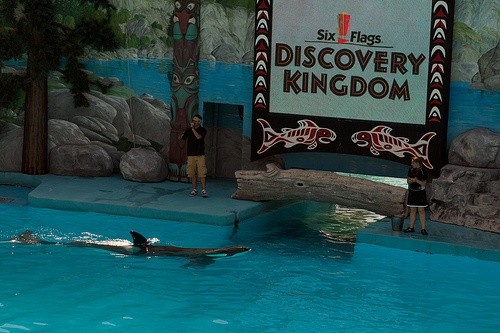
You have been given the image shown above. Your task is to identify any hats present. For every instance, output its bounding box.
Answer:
[412,156,424,165]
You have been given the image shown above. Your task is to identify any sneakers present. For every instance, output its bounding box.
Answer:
[201,189,208,197]
[188,189,198,197]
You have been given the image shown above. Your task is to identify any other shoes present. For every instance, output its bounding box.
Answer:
[404,227,415,232]
[421,229,428,235]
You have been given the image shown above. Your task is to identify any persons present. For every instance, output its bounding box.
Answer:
[183,114,209,198]
[406,157,428,236]
